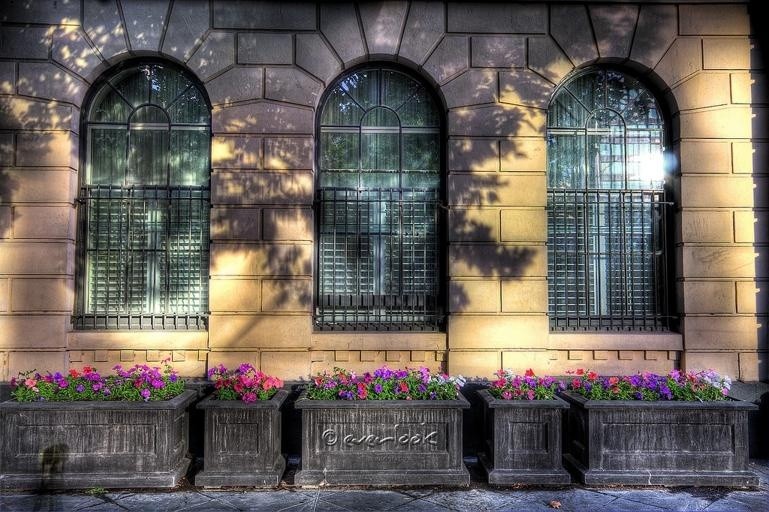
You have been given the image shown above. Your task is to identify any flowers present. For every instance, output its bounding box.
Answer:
[311,363,468,402]
[485,366,565,401]
[206,362,284,400]
[565,366,738,403]
[9,356,179,400]
